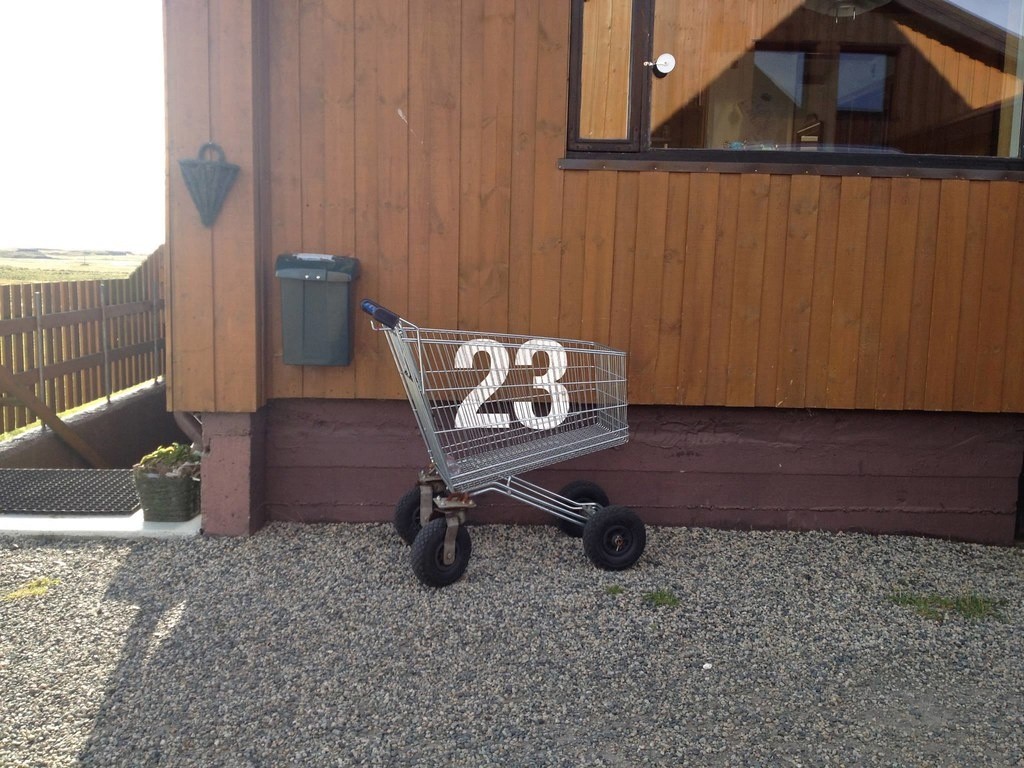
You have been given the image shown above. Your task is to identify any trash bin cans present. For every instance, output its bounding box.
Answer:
[274,252,361,368]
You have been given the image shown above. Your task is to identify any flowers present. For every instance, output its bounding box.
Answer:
[141,442,201,465]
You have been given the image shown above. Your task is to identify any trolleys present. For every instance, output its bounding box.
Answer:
[359,299,647,585]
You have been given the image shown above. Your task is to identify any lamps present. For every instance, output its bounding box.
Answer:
[180,143,240,227]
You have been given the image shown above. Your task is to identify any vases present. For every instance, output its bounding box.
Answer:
[132,464,202,523]
